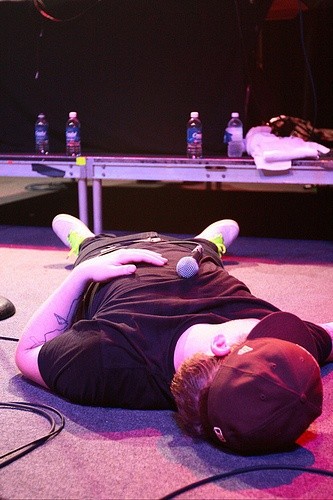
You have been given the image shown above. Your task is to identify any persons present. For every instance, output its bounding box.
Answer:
[15,212,333,458]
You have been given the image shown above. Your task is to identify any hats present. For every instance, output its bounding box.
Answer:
[207,312,323,455]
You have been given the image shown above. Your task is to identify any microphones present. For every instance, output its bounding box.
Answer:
[176,245,204,279]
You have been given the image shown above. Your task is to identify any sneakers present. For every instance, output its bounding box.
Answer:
[194,219,240,258]
[52,213,94,255]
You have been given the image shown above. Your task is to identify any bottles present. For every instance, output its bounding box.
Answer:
[226,113,244,158]
[186,111,203,157]
[34,112,48,156]
[65,112,81,156]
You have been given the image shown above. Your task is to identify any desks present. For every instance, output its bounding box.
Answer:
[0,154,333,235]
